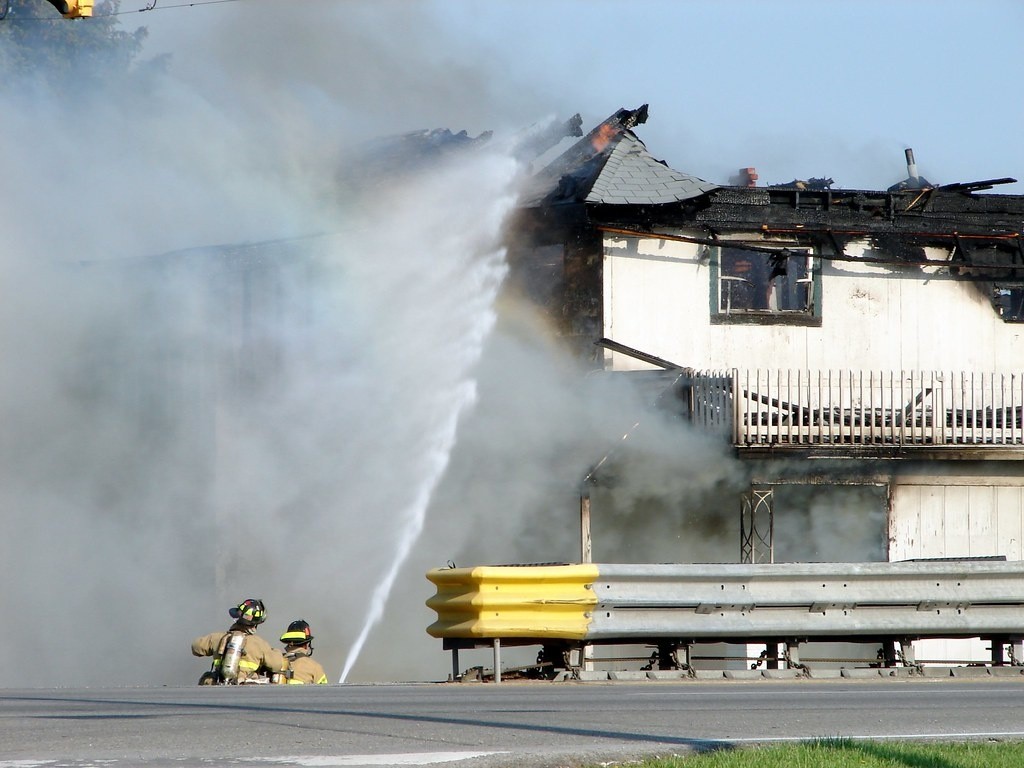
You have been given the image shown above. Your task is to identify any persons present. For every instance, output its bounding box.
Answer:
[191,599,283,685]
[280,620,328,684]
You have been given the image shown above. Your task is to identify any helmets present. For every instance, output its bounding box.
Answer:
[280,620,314,645]
[229,599,266,625]
